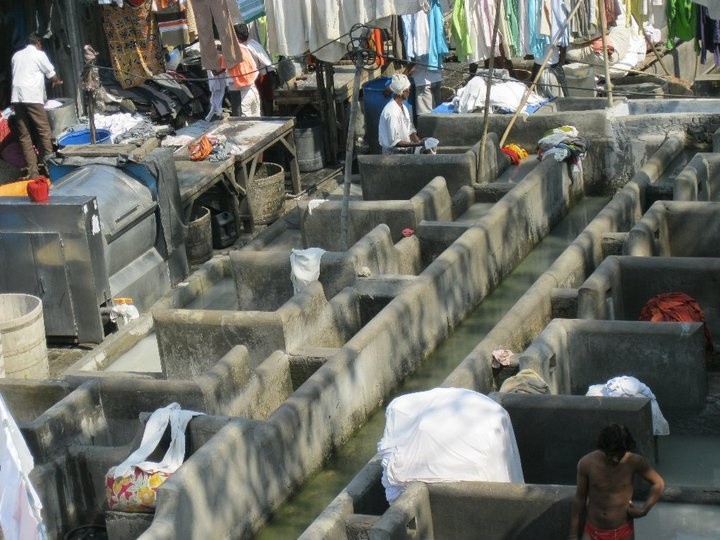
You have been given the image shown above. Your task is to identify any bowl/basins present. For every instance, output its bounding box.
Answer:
[57,128,112,151]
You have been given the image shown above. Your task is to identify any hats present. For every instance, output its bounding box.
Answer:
[389,74,412,95]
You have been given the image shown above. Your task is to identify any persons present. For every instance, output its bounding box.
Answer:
[11,31,64,182]
[569,424,665,540]
[378,41,571,155]
[205,24,277,171]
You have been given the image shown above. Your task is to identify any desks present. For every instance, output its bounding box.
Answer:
[168,115,302,207]
[273,59,381,163]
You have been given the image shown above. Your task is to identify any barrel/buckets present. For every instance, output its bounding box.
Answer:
[362,77,415,145]
[249,161,286,226]
[206,205,237,250]
[183,205,214,267]
[294,118,327,173]
[0,293,50,380]
